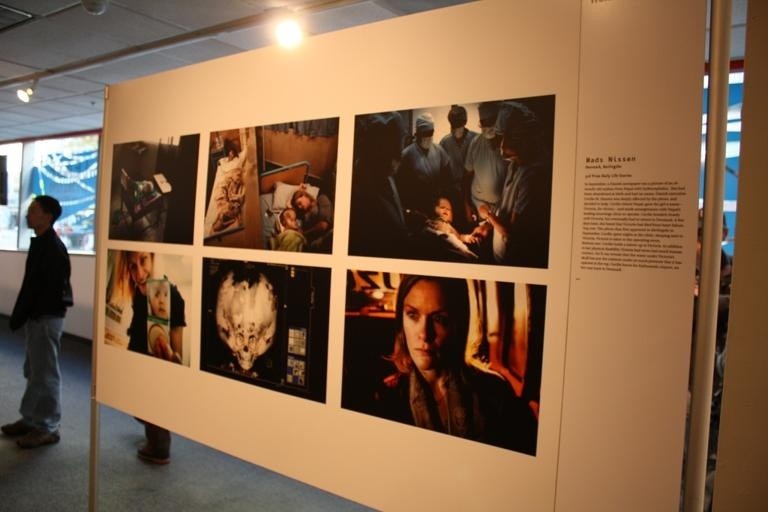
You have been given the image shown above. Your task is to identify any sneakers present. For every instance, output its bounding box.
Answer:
[1,418,60,449]
[136,444,172,466]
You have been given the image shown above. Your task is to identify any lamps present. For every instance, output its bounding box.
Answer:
[13,78,38,104]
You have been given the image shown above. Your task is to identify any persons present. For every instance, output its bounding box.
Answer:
[280,208,305,234]
[286,183,334,254]
[220,149,240,171]
[133,417,170,465]
[366,274,515,448]
[688,208,733,471]
[0,195,73,449]
[148,280,170,320]
[110,250,187,364]
[350,99,552,267]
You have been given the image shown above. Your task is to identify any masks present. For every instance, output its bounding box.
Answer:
[418,136,433,150]
[482,127,497,140]
[393,137,401,161]
[454,127,465,139]
